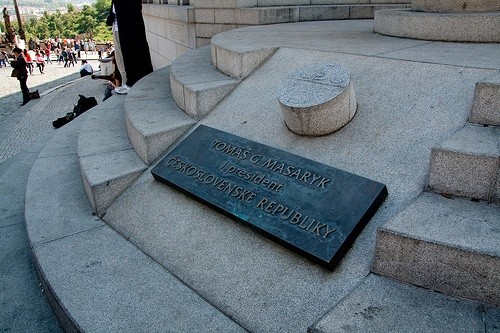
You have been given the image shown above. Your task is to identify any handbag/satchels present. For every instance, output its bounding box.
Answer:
[26,89,40,99]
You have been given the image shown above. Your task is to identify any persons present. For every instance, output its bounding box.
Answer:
[0,0,154,102]
[9,47,31,106]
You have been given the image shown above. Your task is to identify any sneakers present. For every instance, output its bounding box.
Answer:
[114,83,130,95]
